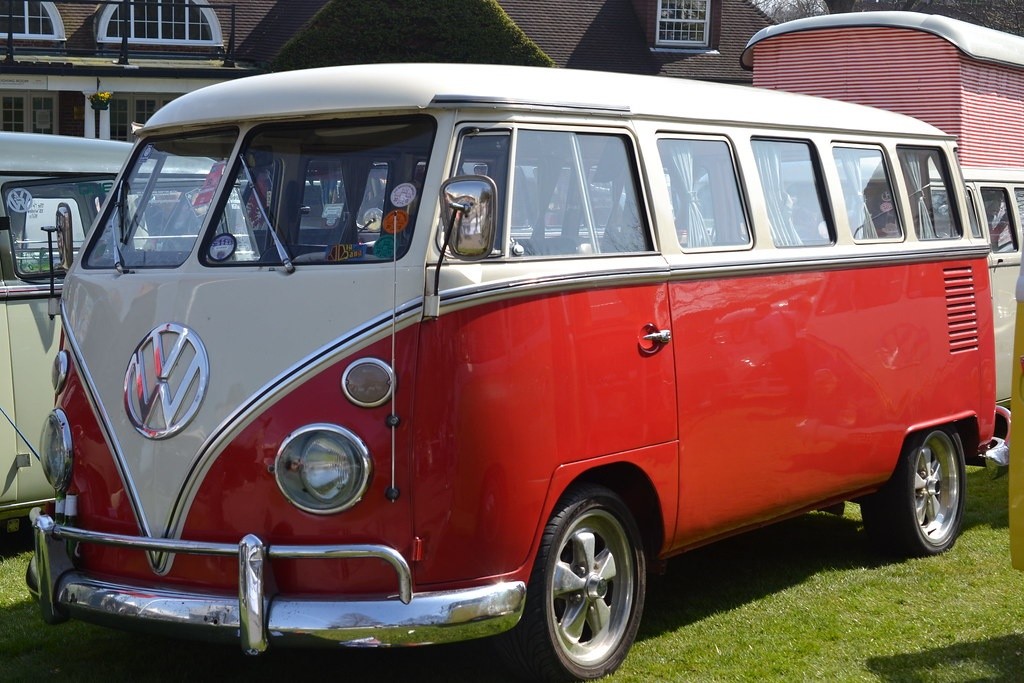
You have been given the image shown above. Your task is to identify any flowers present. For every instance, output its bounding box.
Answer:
[88,91,112,105]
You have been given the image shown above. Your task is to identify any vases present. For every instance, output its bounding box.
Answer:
[91,103,108,110]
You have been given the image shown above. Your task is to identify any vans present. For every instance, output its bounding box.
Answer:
[25,61,1012,683]
[0,129,268,525]
[743,10,1024,431]
